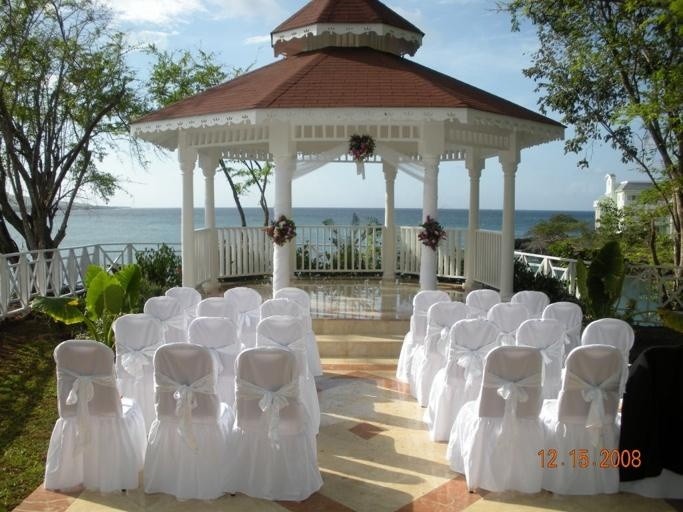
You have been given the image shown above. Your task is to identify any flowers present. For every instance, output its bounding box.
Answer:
[263,213,295,245]
[417,215,446,250]
[349,133,375,161]
